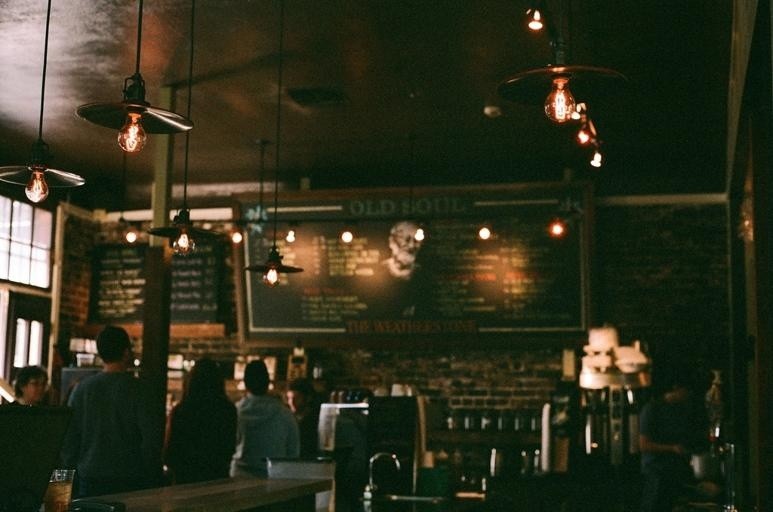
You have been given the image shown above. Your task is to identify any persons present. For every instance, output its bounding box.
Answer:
[227,357,301,479]
[163,354,236,486]
[379,217,425,278]
[285,373,322,462]
[8,364,50,408]
[67,324,162,504]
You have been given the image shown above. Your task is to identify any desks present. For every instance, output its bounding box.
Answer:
[38,479,332,512]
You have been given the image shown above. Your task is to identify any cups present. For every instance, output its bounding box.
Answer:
[43,469,78,511]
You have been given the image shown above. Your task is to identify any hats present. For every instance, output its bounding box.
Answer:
[94,323,134,351]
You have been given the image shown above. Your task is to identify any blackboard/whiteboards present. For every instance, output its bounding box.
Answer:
[93,217,228,339]
[238,207,587,346]
[56,210,95,340]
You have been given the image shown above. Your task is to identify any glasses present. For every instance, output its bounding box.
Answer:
[30,381,49,391]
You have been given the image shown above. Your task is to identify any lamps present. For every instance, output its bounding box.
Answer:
[527,8,605,167]
[244,0,303,284]
[0,0,87,202]
[500,0,614,124]
[76,0,194,152]
[117,212,572,241]
[148,0,213,257]
